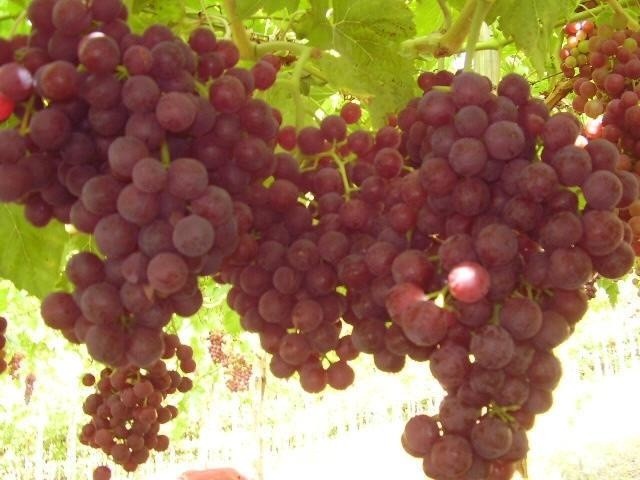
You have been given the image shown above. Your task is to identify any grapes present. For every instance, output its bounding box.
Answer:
[0,0,640,479]
